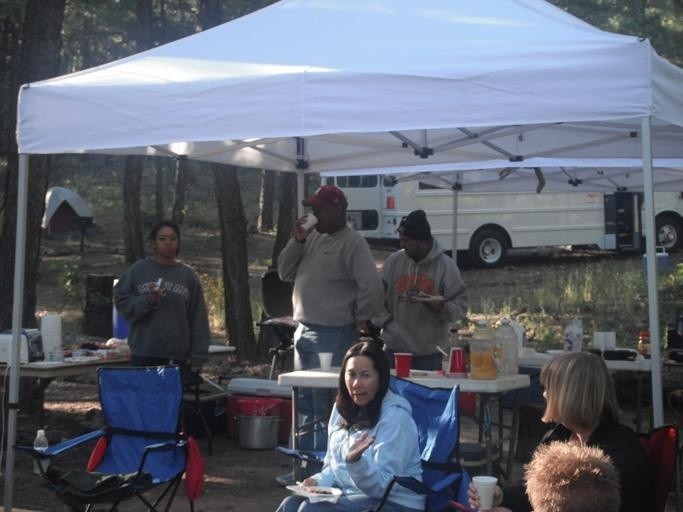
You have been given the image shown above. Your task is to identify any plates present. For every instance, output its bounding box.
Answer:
[401,293,449,302]
[285,485,343,504]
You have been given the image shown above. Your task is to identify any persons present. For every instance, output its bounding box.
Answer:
[112,223,210,382]
[469,353,662,512]
[523,440,621,512]
[374,211,468,371]
[276,186,385,489]
[277,343,424,512]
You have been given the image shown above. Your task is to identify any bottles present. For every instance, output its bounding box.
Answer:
[572,315,583,355]
[563,315,575,353]
[33,428,49,475]
[496,319,519,380]
[638,331,652,359]
[447,328,459,351]
[470,320,498,379]
[512,316,523,358]
[459,335,475,373]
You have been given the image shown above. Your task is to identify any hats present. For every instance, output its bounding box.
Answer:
[396,208,434,244]
[301,184,350,213]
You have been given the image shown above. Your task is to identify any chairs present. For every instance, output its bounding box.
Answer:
[30,361,208,512]
[613,422,680,512]
[274,373,465,512]
[256,268,302,379]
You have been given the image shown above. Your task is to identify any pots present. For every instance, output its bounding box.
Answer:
[234,414,281,450]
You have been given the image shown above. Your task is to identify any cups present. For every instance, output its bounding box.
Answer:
[472,476,498,511]
[152,278,165,297]
[442,355,449,373]
[448,348,467,378]
[299,213,319,231]
[319,352,333,371]
[40,312,62,360]
[394,353,413,378]
